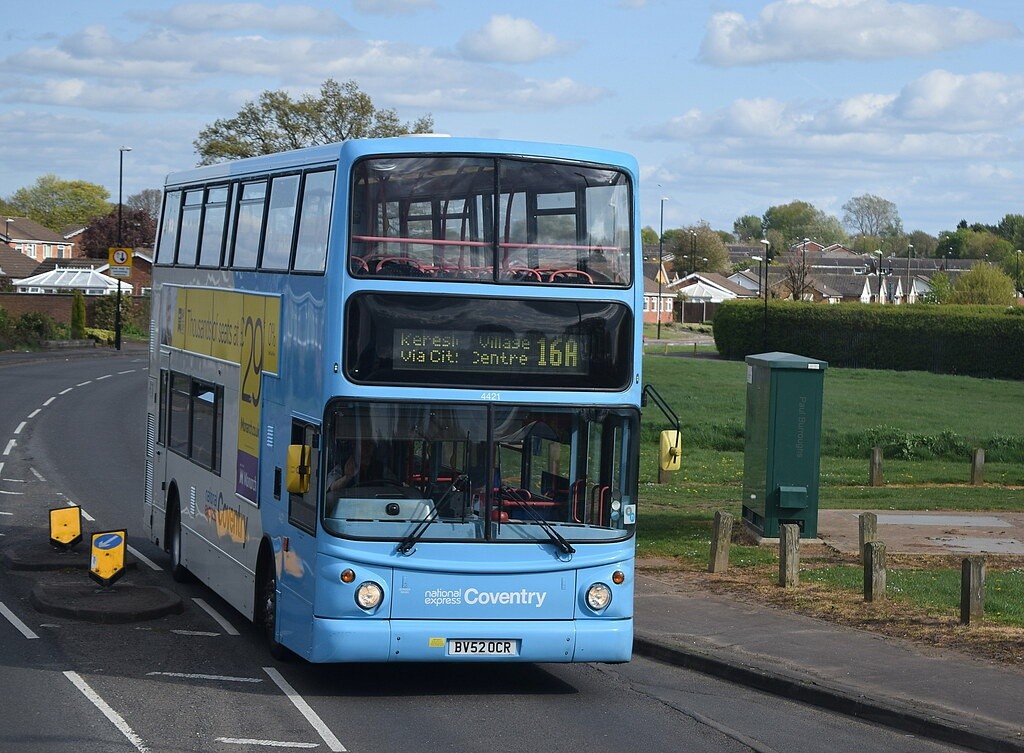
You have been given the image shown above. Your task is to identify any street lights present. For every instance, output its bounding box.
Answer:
[802,238,810,301]
[5,218,14,245]
[1016,249,1021,292]
[752,255,763,298]
[875,249,884,302]
[608,203,616,261]
[658,197,670,339]
[690,229,697,272]
[114,147,133,350]
[760,239,770,352]
[946,235,950,270]
[907,243,913,295]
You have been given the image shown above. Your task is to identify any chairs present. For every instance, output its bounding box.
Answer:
[350,253,594,284]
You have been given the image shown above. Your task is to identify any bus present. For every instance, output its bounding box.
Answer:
[143,133,681,669]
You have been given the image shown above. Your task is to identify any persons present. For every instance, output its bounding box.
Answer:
[326,427,410,492]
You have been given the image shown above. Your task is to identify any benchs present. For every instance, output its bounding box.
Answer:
[473,488,519,504]
[415,477,463,491]
[504,501,561,520]
